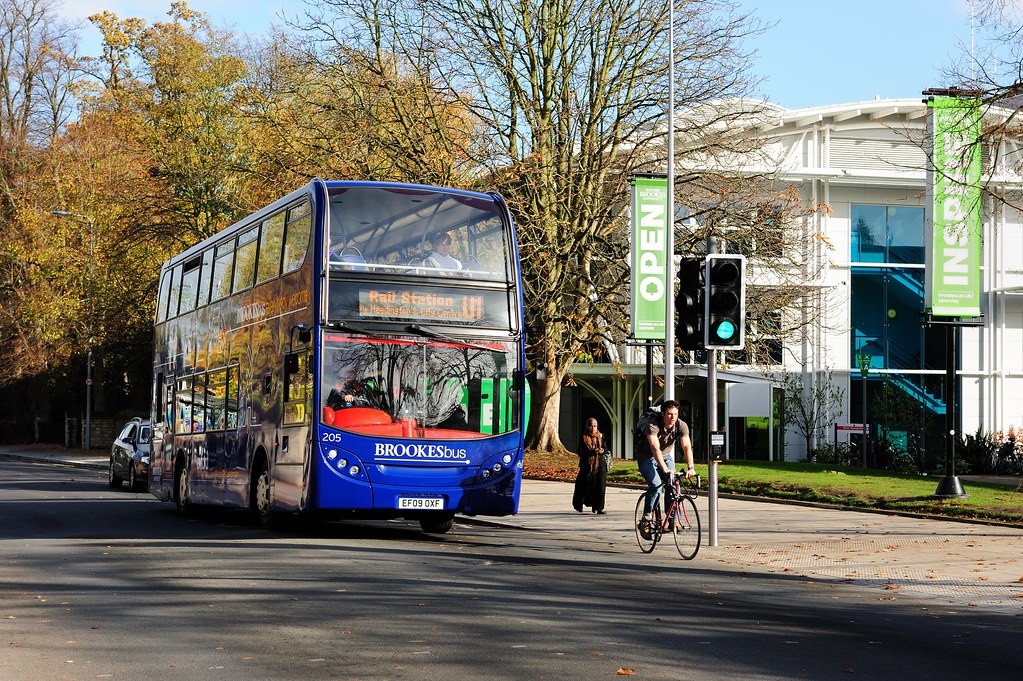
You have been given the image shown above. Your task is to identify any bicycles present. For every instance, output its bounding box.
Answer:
[635,467,702,560]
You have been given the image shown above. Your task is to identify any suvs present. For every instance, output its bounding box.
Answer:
[108,417,151,491]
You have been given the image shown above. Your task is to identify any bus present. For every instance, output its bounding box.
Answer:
[147,177,550,537]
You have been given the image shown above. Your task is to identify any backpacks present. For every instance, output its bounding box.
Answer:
[634,405,679,452]
[603,450,614,472]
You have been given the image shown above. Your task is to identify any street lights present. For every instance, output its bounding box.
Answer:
[51,208,96,455]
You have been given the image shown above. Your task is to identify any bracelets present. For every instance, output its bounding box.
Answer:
[688,467,694,470]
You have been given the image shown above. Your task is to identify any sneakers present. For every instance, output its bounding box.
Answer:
[668,517,683,534]
[639,518,653,540]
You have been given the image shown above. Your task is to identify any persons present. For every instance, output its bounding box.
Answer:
[419,232,465,270]
[325,364,378,413]
[185,420,201,433]
[914,344,928,390]
[636,400,697,541]
[295,235,344,272]
[572,417,608,514]
[217,418,225,430]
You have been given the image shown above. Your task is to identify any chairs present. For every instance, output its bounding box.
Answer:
[340,247,369,272]
[458,254,485,272]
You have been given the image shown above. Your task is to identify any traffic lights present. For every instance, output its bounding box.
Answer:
[704,253,746,351]
[676,257,704,351]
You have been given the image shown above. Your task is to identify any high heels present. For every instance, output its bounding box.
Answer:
[574,505,582,512]
[591,507,606,515]
